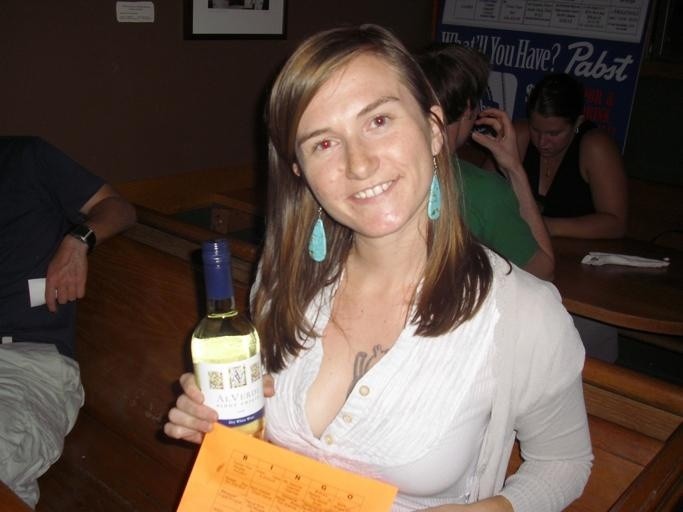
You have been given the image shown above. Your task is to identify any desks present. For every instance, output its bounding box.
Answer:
[544,238,681,337]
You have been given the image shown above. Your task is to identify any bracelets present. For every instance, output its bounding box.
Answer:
[60,218,96,257]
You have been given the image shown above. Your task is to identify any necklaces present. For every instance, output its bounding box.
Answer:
[539,153,561,181]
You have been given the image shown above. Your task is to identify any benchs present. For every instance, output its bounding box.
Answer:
[40,209,681,512]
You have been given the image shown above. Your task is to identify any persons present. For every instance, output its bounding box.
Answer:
[481,72,631,242]
[426,43,557,280]
[163,24,600,510]
[0,132,137,510]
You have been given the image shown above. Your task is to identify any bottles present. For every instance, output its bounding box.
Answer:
[190,237,267,442]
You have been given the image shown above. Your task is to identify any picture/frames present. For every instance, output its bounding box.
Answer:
[183,0,288,42]
